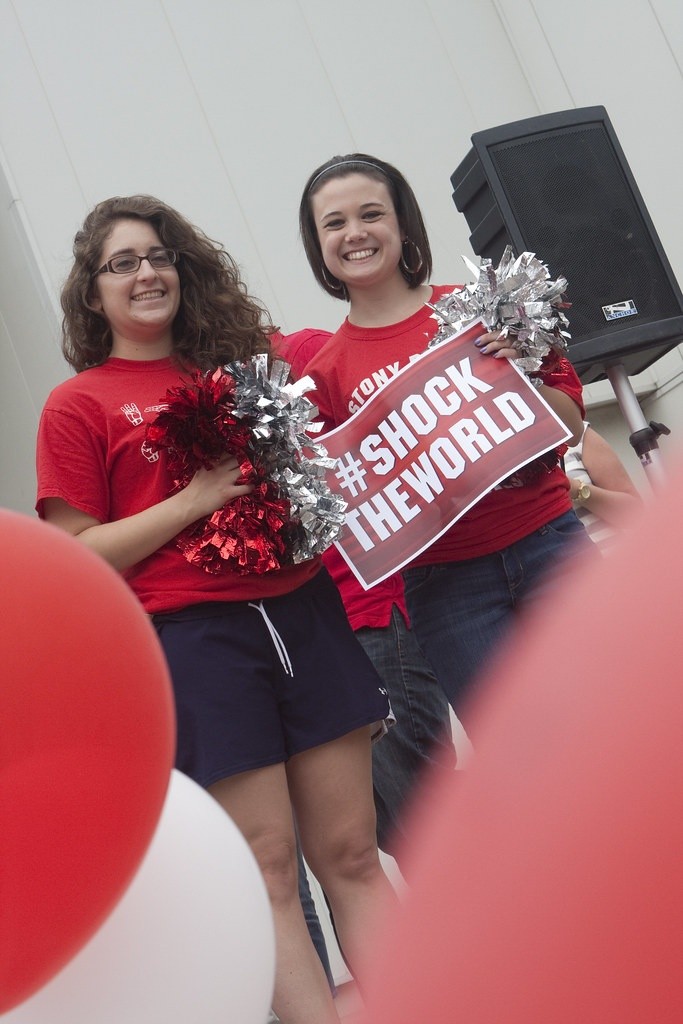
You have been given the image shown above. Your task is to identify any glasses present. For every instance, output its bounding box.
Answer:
[89,247,179,278]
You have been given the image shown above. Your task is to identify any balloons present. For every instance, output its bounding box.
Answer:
[3,506,279,1024]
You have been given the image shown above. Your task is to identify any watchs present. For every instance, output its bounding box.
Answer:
[570,478,594,504]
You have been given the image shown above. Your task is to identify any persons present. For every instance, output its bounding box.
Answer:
[253,156,641,1005]
[36,195,408,1024]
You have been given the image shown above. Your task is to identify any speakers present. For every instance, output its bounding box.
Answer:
[450,105,683,388]
